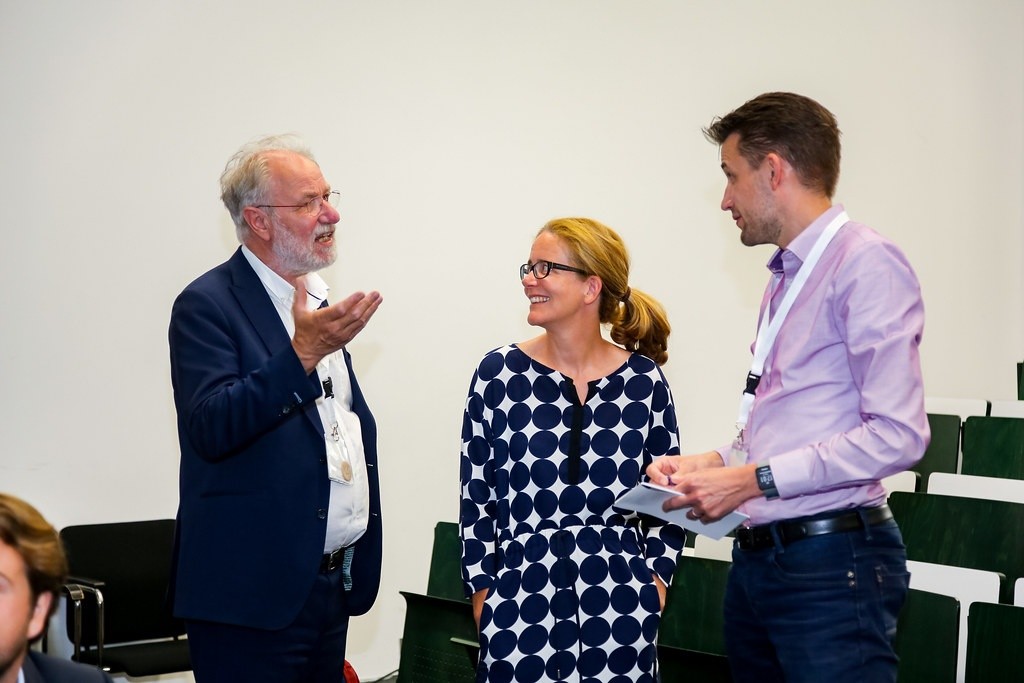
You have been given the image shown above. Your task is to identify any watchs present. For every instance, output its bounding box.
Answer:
[756,459,778,498]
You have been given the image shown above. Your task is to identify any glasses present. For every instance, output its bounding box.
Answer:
[520,260,596,279]
[255,189,343,217]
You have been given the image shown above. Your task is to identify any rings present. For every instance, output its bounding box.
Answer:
[692,509,702,518]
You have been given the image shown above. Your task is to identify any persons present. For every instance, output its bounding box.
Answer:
[168,134,383,683]
[458,217,688,683]
[647,92,932,683]
[0,494,113,683]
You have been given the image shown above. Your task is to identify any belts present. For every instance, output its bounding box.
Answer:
[320,547,345,571]
[738,503,893,550]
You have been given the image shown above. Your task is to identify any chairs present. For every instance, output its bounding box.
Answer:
[392,362,1024,683]
[60,518,193,676]
